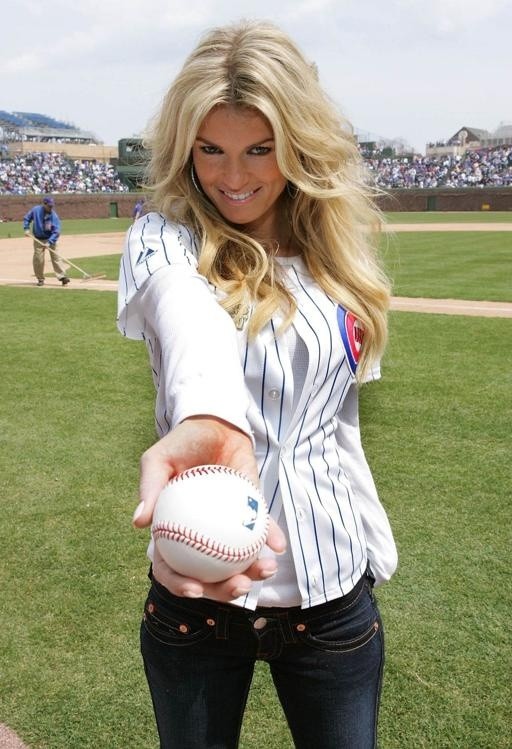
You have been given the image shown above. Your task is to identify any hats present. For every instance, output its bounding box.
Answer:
[43,197,55,207]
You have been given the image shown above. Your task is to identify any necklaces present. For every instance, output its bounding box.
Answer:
[280,202,295,257]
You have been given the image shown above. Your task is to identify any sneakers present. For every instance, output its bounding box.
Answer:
[63,278,70,284]
[38,281,44,286]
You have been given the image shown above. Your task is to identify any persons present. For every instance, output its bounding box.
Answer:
[116,22,394,749]
[358,142,512,190]
[132,197,144,221]
[2,142,128,198]
[21,197,70,289]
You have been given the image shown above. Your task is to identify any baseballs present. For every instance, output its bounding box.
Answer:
[152,464,269,583]
